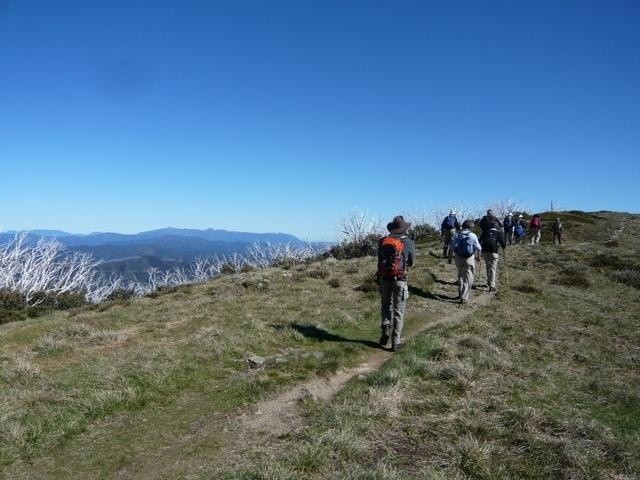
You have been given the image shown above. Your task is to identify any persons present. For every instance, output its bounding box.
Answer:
[530,213,542,245]
[480,209,503,233]
[440,209,461,264]
[502,211,516,245]
[514,214,526,243]
[377,214,416,352]
[447,219,483,306]
[551,217,564,245]
[477,221,506,293]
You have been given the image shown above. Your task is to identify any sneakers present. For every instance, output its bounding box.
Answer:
[380,325,390,345]
[391,340,406,352]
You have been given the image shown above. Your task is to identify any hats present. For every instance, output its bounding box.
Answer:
[386,216,411,234]
[449,209,456,214]
[509,213,523,218]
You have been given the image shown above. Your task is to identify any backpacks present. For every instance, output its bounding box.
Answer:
[445,215,498,258]
[378,234,411,276]
